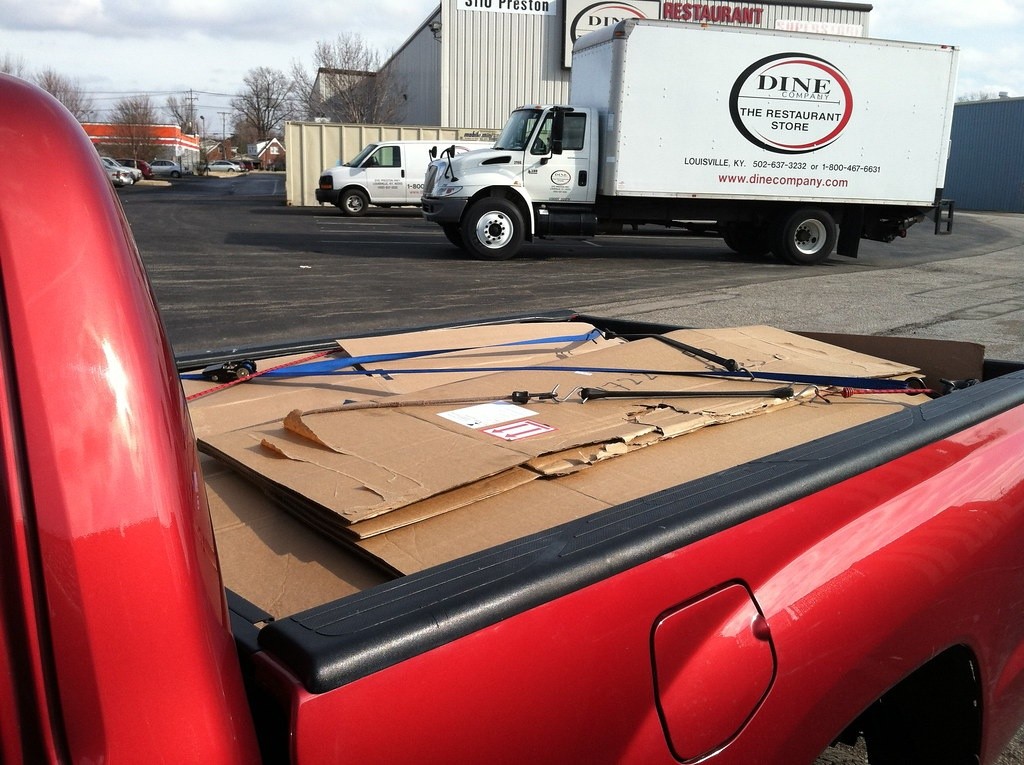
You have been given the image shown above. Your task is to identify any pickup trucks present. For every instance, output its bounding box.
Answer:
[0,73,1024,765]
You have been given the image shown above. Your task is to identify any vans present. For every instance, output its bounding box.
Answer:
[315,140,497,216]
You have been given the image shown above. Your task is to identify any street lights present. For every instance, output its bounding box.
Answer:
[200,116,206,156]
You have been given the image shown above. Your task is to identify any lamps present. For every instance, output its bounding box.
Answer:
[429,22,442,43]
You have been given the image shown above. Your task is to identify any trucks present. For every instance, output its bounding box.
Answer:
[422,17,961,266]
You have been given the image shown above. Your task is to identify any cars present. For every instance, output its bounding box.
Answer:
[149,160,186,178]
[100,157,142,188]
[204,158,254,172]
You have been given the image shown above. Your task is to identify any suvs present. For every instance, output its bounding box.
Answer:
[116,159,155,180]
